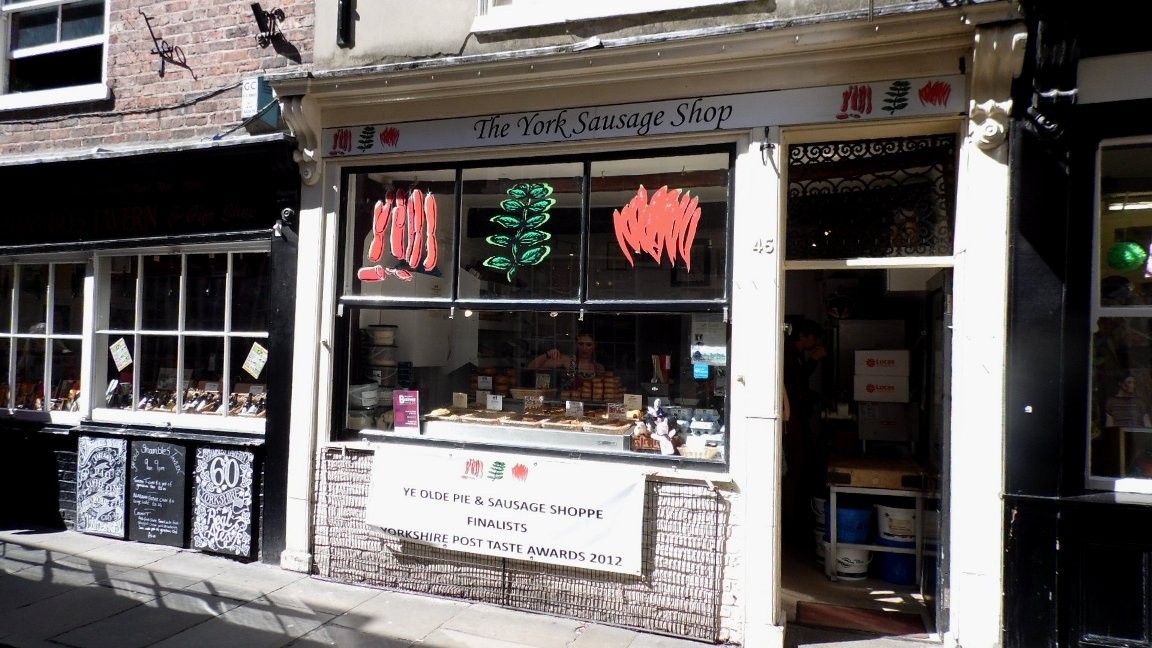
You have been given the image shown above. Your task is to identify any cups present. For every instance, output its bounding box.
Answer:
[120,383,130,394]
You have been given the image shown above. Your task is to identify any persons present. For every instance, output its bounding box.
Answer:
[525,332,606,390]
[781,319,827,478]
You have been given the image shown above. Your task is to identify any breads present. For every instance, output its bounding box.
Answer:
[522,405,642,427]
[560,371,628,400]
[484,367,517,392]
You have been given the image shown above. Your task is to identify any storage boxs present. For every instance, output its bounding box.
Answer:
[347,325,399,432]
[826,318,933,493]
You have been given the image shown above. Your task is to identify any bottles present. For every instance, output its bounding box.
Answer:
[650,358,663,384]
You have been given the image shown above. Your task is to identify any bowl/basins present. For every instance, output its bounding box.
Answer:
[50,399,64,411]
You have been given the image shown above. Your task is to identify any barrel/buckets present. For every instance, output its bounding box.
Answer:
[823,542,870,581]
[367,366,398,387]
[379,385,401,406]
[811,497,827,558]
[367,325,399,346]
[876,504,938,592]
[349,379,379,409]
[368,345,400,367]
[348,408,380,430]
[837,508,872,544]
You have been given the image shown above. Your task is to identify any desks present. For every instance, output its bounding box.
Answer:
[828,486,942,585]
